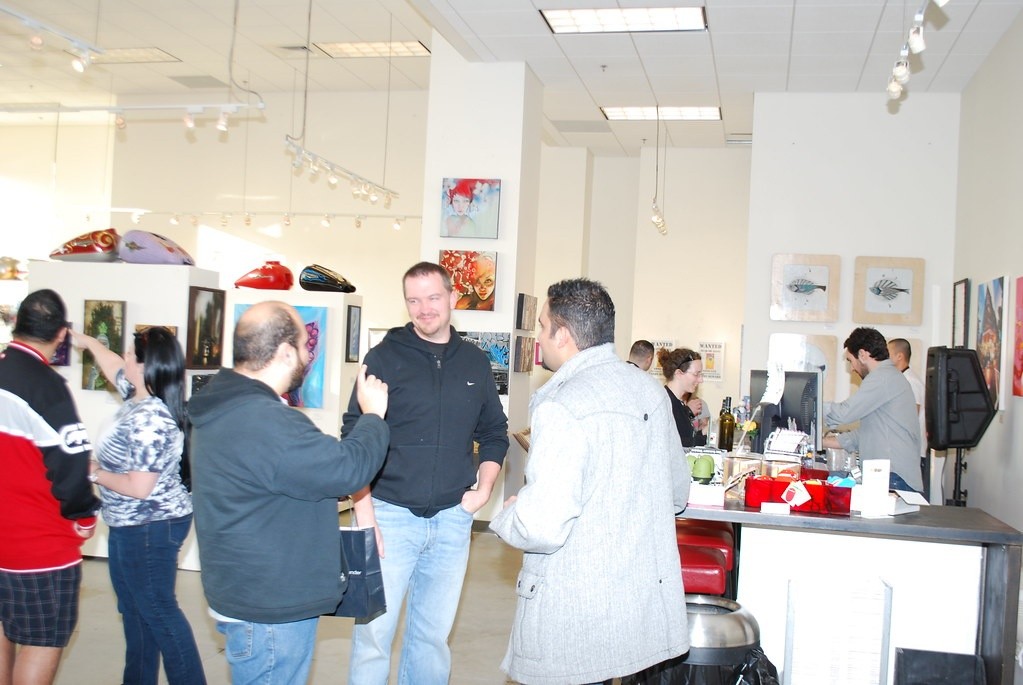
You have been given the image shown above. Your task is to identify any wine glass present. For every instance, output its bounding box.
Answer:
[718,397,734,452]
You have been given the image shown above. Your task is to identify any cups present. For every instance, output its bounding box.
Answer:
[827,447,844,471]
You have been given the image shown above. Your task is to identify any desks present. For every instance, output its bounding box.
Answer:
[668,503,1023,685]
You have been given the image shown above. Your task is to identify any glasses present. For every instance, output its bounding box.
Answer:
[674,352,701,372]
[137,326,149,347]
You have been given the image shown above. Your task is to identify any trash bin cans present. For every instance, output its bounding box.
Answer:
[622,595,759,685]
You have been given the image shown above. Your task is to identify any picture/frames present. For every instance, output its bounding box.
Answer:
[770,252,841,322]
[346,304,361,364]
[81,299,127,391]
[851,257,924,326]
[186,286,226,371]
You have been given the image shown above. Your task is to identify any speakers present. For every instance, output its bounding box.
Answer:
[925,346,996,452]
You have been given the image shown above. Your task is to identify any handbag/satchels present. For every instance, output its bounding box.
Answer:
[321,498,388,627]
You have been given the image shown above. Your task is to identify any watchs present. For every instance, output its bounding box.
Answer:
[88,467,101,484]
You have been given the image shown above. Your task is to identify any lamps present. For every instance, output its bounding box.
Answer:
[183,110,197,131]
[25,17,44,53]
[115,112,127,133]
[888,12,926,100]
[285,135,400,208]
[215,108,234,133]
[68,48,99,76]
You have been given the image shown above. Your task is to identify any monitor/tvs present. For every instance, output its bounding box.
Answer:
[749,369,823,454]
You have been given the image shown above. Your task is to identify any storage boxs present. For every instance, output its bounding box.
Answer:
[850,486,931,516]
[744,474,852,516]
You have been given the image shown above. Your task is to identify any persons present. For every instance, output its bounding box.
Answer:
[0,287,102,685]
[820,328,925,498]
[488,278,690,685]
[66,325,207,685]
[887,338,927,487]
[627,340,710,448]
[188,300,390,684]
[342,261,510,685]
